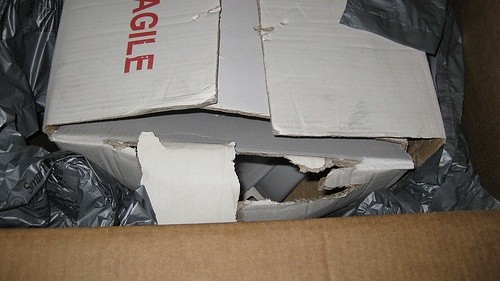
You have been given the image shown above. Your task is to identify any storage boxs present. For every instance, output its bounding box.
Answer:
[42,0,447,226]
